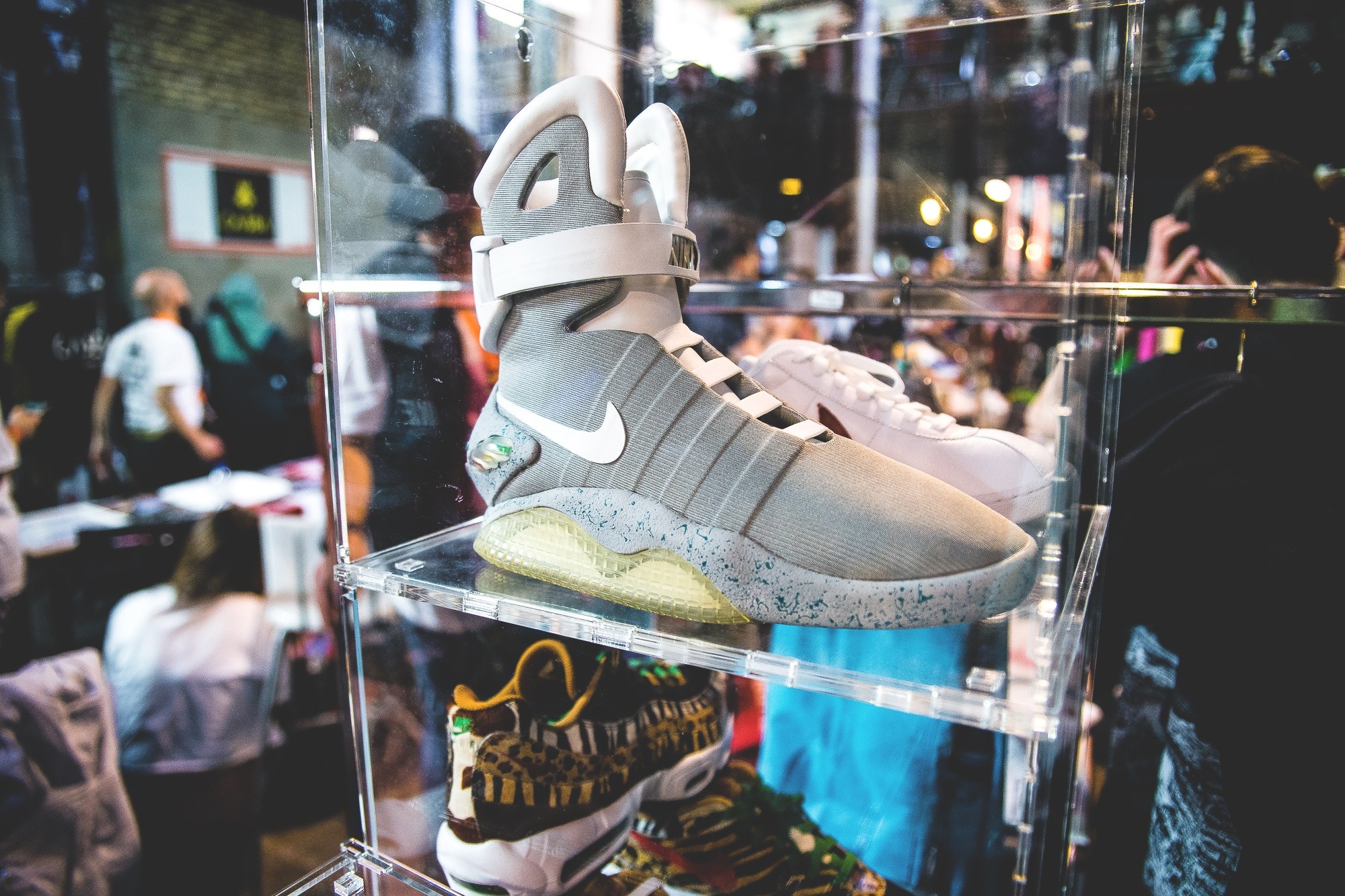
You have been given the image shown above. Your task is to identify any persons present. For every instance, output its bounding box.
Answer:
[0,117,1345,896]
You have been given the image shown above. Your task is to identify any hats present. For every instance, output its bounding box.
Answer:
[388,115,481,225]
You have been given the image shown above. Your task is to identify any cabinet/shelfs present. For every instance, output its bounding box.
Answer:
[304,0,1147,896]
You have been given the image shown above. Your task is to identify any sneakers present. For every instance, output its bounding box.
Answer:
[610,758,916,896]
[472,76,1040,626]
[745,341,1058,525]
[437,639,737,896]
